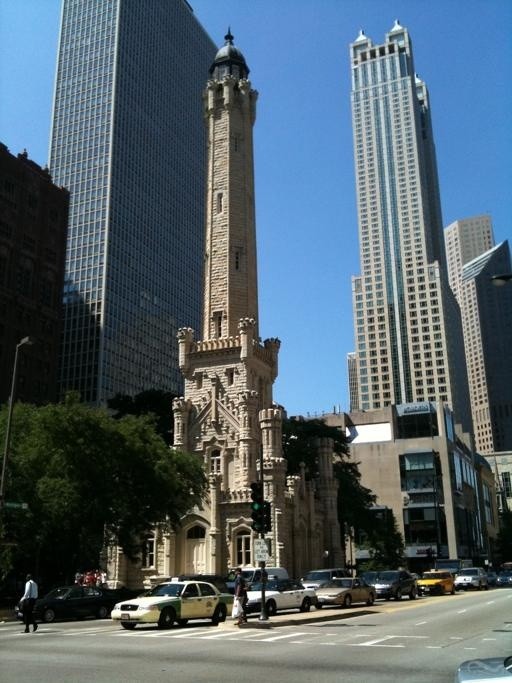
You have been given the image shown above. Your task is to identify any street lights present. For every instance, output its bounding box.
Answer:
[490,273,512,286]
[1,335,38,498]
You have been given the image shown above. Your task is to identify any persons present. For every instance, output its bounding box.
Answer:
[243,581,248,622]
[74,567,108,588]
[19,573,38,633]
[232,567,245,624]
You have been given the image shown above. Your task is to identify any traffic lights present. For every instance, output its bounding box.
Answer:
[250,481,264,532]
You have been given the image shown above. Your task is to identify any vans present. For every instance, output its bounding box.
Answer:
[225,566,289,594]
[299,568,352,591]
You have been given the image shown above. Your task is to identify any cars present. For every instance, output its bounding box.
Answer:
[164,575,229,594]
[245,579,318,615]
[111,580,236,630]
[316,577,377,608]
[356,557,511,601]
[14,586,116,622]
[455,654,512,683]
